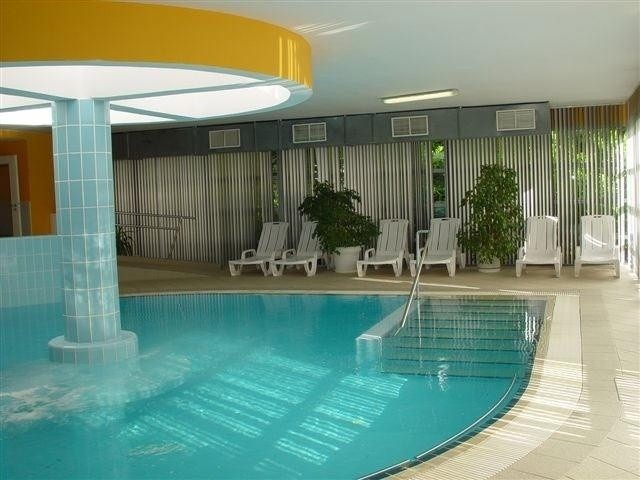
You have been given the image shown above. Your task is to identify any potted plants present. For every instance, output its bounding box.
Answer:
[297,174,381,274]
[454,161,527,273]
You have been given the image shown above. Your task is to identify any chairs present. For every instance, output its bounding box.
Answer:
[355,217,461,279]
[227,219,333,278]
[573,213,622,278]
[514,214,566,278]
